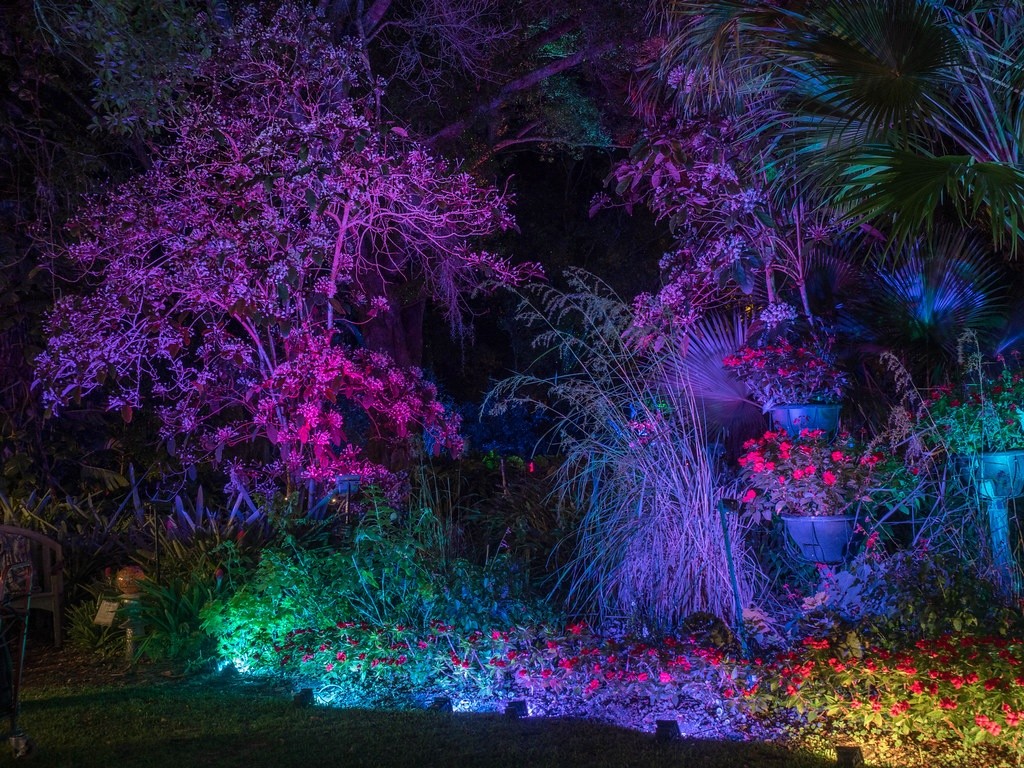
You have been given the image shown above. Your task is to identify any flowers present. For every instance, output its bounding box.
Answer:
[737,426,885,517]
[720,335,854,415]
[916,348,1024,455]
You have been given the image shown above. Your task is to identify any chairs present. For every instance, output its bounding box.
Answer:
[0,524,76,649]
[0,562,36,738]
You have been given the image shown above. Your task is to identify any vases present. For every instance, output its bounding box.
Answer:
[780,513,855,563]
[769,403,843,437]
[952,450,1024,499]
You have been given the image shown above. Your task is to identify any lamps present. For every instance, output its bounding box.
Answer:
[115,565,146,605]
[292,687,316,709]
[426,697,453,715]
[505,698,529,720]
[654,719,681,744]
[835,746,864,768]
